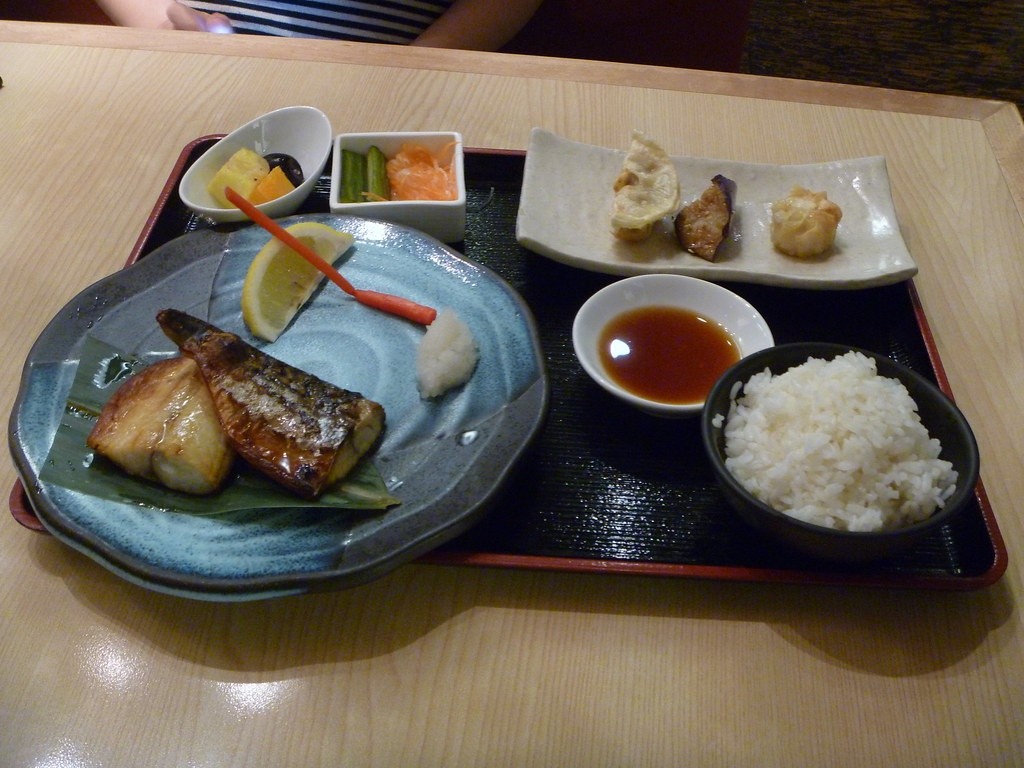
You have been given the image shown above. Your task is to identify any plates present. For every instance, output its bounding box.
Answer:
[8,214,548,605]
[571,274,776,411]
[515,126,919,287]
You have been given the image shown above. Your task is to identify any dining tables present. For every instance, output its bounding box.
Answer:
[0,19,1024,768]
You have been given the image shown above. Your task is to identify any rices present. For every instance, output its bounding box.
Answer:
[712,349,958,532]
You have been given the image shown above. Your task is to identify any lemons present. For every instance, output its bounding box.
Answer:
[242,222,354,342]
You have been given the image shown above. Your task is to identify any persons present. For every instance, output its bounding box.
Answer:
[91,0,541,53]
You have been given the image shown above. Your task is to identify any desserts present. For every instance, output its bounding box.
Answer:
[608,133,680,241]
[770,185,841,258]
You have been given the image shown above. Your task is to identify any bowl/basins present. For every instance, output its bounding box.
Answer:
[178,105,332,220]
[703,341,980,543]
[329,131,467,243]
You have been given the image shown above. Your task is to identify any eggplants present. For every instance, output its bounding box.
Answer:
[674,174,737,263]
